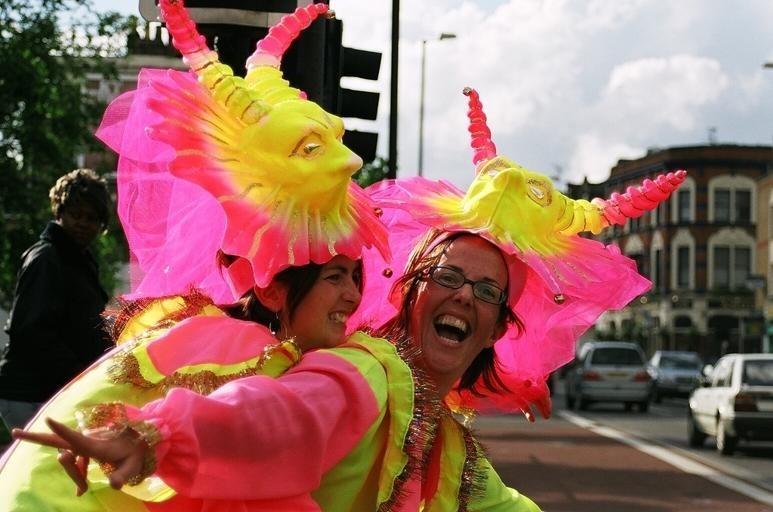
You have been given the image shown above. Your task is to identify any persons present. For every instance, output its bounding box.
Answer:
[1,167,118,444]
[9,223,549,511]
[2,239,361,510]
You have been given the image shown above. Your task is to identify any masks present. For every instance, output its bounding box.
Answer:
[68,223,98,250]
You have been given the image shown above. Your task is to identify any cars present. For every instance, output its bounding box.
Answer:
[685,352,772,457]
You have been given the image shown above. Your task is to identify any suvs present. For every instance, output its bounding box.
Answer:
[646,351,709,409]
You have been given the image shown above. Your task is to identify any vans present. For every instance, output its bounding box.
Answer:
[563,339,652,413]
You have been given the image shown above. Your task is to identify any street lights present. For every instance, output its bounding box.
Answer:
[416,31,457,178]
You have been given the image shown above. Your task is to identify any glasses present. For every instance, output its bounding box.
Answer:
[418,261,509,306]
